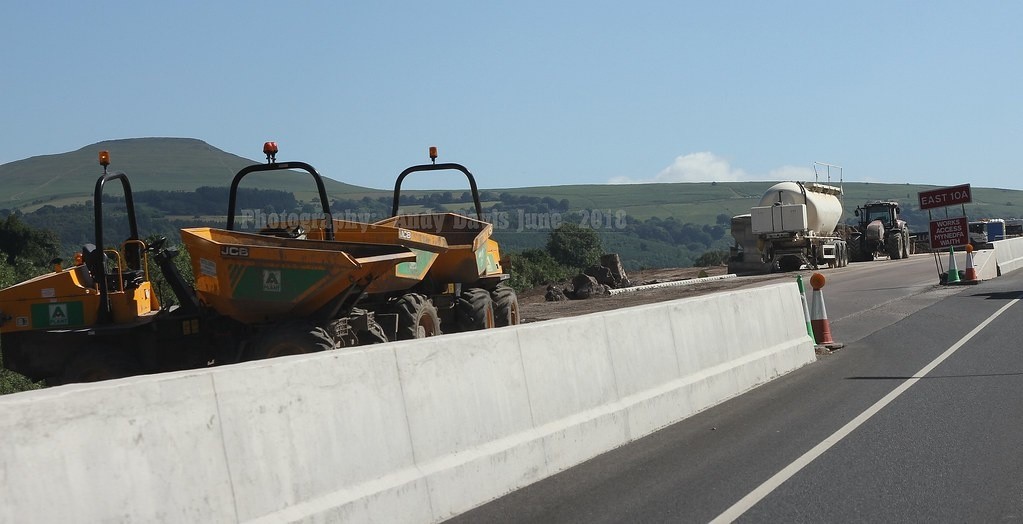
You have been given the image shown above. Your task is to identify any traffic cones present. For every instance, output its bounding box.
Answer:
[960,244,981,283]
[807,272,845,350]
[946,246,960,284]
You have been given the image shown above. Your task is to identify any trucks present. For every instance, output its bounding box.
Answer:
[968,223,989,246]
[1005,218,1023,238]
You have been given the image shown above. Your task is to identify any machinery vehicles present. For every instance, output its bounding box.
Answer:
[847,201,910,261]
[728,214,776,275]
[0,142,521,376]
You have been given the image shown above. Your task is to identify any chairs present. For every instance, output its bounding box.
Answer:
[876,215,887,224]
[82,244,144,281]
